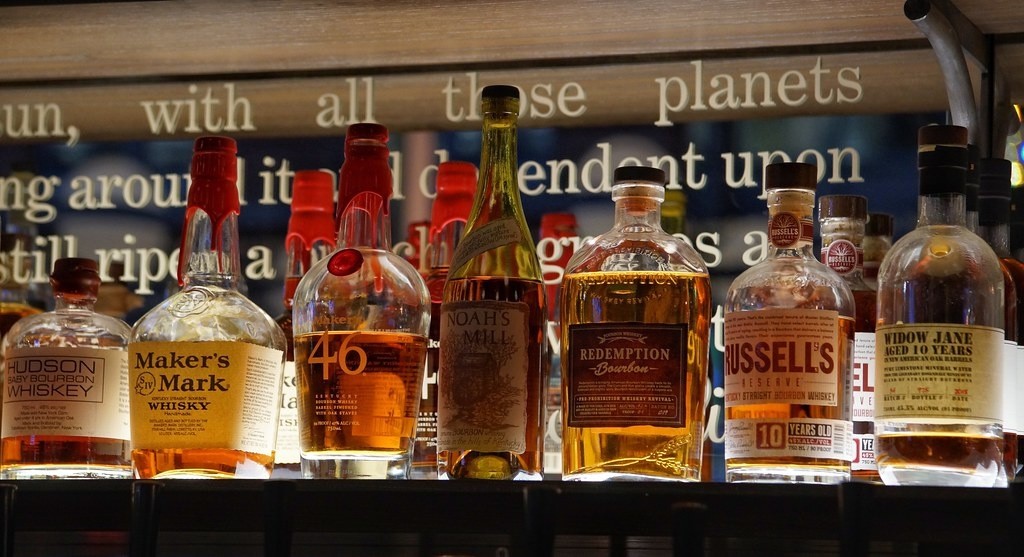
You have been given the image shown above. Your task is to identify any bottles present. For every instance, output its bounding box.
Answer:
[0,85,1024,488]
